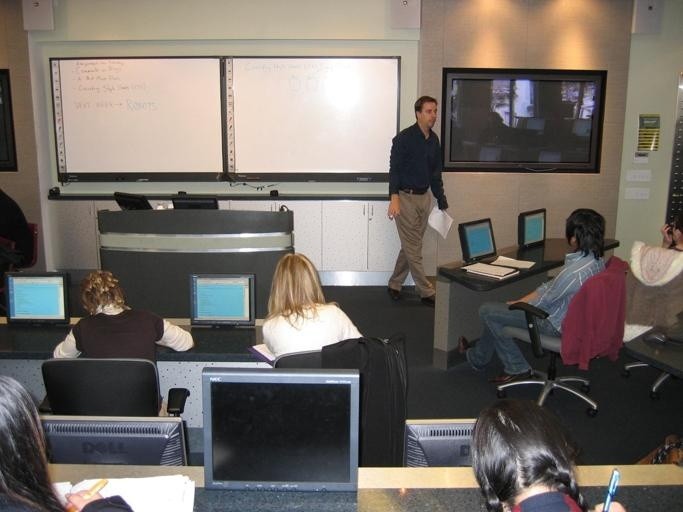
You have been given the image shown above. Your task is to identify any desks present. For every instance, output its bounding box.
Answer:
[0,323,261,454]
[434,237,618,374]
[0,463,682,511]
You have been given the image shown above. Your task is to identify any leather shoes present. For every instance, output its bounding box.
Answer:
[421,293,434,306]
[487,369,531,385]
[458,336,482,376]
[388,288,399,300]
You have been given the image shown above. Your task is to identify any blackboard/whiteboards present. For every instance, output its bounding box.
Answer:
[42,41,420,200]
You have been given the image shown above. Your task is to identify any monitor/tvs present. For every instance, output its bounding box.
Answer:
[402,419,477,468]
[202,367,360,493]
[518,208,546,249]
[172,196,218,209]
[444,72,603,173]
[114,192,154,209]
[4,271,70,324]
[458,218,497,266]
[189,273,255,326]
[0,72,16,169]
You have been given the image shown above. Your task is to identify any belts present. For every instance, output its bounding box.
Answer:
[399,184,429,194]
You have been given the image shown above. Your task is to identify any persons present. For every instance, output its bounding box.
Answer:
[1,184,36,317]
[261,252,366,358]
[663,213,683,258]
[51,266,197,411]
[0,373,136,512]
[385,94,452,308]
[458,207,610,386]
[471,396,625,512]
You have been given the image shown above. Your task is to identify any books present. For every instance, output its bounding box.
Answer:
[458,261,521,280]
[248,338,275,364]
[51,472,194,512]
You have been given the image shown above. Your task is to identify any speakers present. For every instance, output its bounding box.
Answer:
[22,0,54,30]
[632,0,664,33]
[390,0,421,28]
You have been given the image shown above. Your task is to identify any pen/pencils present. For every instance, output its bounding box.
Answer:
[603,469,620,512]
[68,478,108,512]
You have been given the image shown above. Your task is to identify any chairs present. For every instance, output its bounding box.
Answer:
[622,242,682,398]
[39,357,188,416]
[1,221,37,274]
[496,257,627,415]
[273,335,408,467]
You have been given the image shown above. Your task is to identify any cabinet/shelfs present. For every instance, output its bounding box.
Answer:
[321,198,421,287]
[45,200,121,284]
[229,200,322,285]
